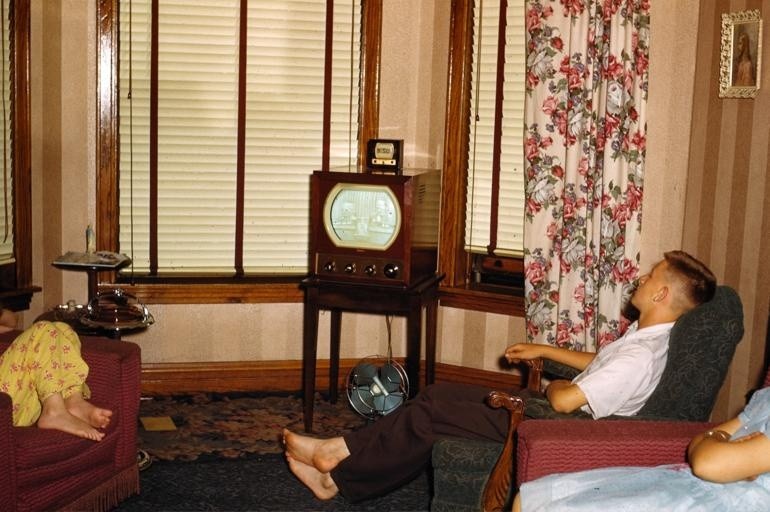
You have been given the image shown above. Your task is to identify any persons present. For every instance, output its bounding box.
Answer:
[0,306,116,446]
[504,378,769,510]
[279,245,716,505]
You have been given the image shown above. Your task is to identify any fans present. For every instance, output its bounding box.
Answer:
[345,354,410,426]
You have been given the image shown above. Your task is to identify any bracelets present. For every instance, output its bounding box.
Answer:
[714,430,729,440]
[704,428,714,438]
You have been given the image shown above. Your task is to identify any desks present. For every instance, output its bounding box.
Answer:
[297,271,447,433]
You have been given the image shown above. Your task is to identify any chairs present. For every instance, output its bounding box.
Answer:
[0,329,142,512]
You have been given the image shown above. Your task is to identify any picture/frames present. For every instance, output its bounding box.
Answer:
[718,9,764,100]
